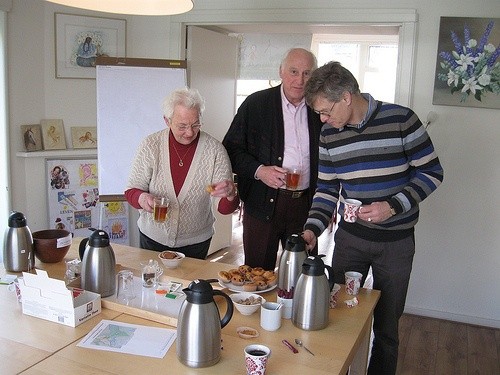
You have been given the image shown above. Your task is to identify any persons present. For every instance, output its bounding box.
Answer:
[300,62,443,375]
[220,47,320,271]
[124,87,238,262]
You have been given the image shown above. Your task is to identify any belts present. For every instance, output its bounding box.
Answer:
[277,188,313,200]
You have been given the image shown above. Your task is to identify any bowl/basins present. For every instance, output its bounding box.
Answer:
[158,251,186,269]
[32,229,73,264]
[229,292,265,315]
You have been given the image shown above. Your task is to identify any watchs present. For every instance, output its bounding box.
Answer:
[387,201,396,216]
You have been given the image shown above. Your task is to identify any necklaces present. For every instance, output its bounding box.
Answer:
[170,136,191,167]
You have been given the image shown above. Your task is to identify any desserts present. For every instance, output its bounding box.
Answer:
[217,265,277,292]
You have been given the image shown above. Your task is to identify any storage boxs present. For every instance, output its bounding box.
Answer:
[16,269,101,328]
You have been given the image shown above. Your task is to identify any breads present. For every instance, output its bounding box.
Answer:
[206,185,216,192]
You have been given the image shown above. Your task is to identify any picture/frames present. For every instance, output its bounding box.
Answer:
[19,124,43,152]
[54,12,127,79]
[70,126,98,149]
[42,119,66,150]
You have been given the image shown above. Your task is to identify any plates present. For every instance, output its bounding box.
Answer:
[236,326,259,338]
[218,277,278,294]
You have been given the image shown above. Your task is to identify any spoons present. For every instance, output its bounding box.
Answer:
[294,338,315,356]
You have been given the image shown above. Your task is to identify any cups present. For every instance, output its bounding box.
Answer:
[65,259,80,282]
[139,258,158,288]
[14,276,24,302]
[282,167,302,188]
[116,269,134,299]
[344,199,363,223]
[344,271,362,295]
[260,302,282,330]
[153,197,170,222]
[244,344,270,375]
[276,295,294,320]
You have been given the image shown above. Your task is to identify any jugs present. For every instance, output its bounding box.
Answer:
[79,227,117,297]
[291,253,335,331]
[176,278,234,368]
[2,210,35,273]
[277,233,312,293]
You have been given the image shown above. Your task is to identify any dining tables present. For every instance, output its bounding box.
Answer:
[0,237,380,375]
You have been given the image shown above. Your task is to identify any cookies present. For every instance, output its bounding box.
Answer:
[160,251,182,259]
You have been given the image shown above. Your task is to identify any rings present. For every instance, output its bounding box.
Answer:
[368,218,371,221]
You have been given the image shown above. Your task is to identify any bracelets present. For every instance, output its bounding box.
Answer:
[234,187,238,196]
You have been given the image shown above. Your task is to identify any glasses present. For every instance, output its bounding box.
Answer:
[168,118,202,132]
[312,89,352,117]
